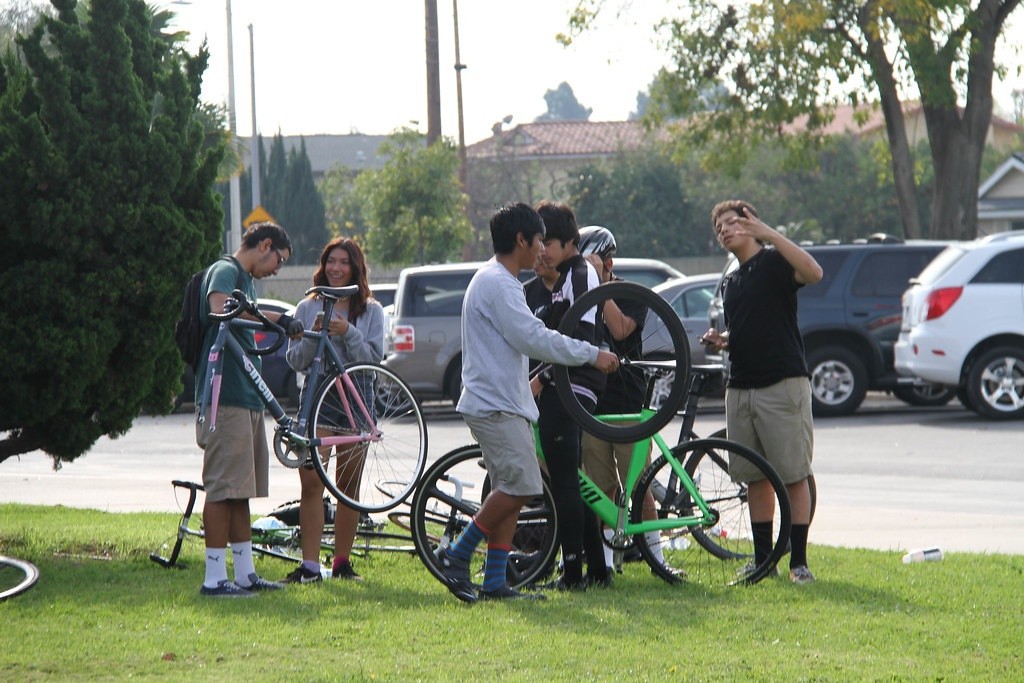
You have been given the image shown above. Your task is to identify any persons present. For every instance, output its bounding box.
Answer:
[193,223,304,596]
[514,198,687,588]
[280,237,383,585]
[700,200,824,584]
[436,201,619,603]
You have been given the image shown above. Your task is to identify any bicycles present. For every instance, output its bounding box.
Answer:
[374,365,792,594]
[149,480,438,568]
[199,285,428,513]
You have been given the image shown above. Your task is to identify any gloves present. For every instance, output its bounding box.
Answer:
[278,314,304,341]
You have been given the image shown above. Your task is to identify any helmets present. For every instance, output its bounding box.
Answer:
[577,225,617,258]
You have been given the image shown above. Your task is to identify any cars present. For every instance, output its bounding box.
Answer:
[641,271,724,398]
[366,284,466,360]
[252,298,300,404]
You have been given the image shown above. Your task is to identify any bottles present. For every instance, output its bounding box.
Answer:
[660,536,692,550]
[902,547,944,564]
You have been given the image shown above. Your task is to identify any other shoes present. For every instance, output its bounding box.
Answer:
[650,568,687,580]
[606,567,615,580]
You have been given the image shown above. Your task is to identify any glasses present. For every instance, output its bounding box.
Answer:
[263,236,286,265]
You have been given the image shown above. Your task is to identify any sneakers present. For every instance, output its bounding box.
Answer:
[331,561,364,582]
[199,579,253,599]
[789,564,814,584]
[437,544,478,602]
[582,571,613,591]
[234,573,287,593]
[478,582,547,603]
[737,562,779,578]
[526,575,586,596]
[279,564,323,587]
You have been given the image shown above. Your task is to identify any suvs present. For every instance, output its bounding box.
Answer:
[893,229,1024,422]
[709,232,957,418]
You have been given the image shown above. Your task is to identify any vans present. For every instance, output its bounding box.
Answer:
[383,258,716,409]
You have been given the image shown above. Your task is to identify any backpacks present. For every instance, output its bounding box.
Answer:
[175,256,243,373]
